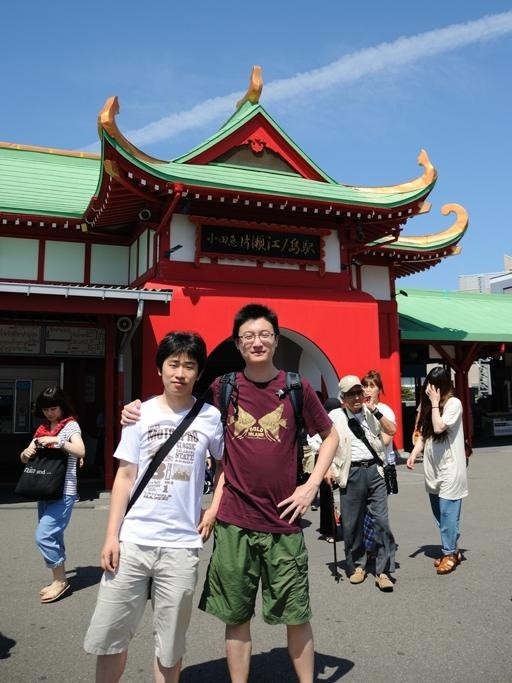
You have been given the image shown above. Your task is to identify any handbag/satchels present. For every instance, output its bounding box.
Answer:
[382,463,399,495]
[14,439,70,503]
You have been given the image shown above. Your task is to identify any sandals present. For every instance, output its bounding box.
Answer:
[40,576,70,603]
[433,550,464,568]
[435,553,459,577]
[40,581,54,596]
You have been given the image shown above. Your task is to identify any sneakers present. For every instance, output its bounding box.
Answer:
[325,536,335,543]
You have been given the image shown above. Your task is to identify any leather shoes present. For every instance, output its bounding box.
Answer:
[349,567,368,584]
[374,571,394,591]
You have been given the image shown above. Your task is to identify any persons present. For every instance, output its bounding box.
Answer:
[359,371,396,558]
[306,396,340,543]
[18,384,86,603]
[327,374,398,593]
[117,302,341,682]
[82,328,226,682]
[406,368,471,573]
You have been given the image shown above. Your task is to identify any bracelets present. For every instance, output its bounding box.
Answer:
[371,407,383,420]
[431,406,440,408]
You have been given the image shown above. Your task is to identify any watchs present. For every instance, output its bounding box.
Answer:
[58,437,65,447]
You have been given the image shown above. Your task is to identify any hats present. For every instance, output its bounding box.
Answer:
[337,375,364,394]
